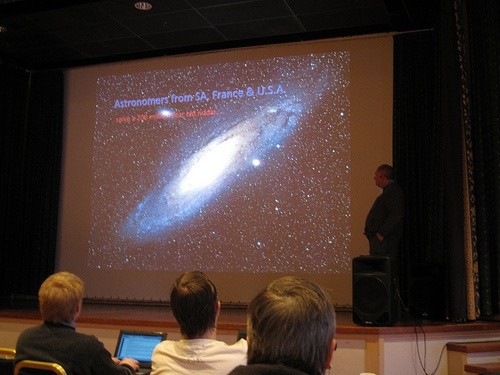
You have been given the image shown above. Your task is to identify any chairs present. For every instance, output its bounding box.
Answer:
[0,353,68,375]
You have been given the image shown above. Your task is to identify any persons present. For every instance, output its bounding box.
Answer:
[363,164,404,301]
[227,276,337,375]
[13,271,140,375]
[150,270,248,375]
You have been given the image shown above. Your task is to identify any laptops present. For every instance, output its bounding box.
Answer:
[114,330,167,375]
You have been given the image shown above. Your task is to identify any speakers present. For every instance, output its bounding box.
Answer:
[352,255,400,325]
